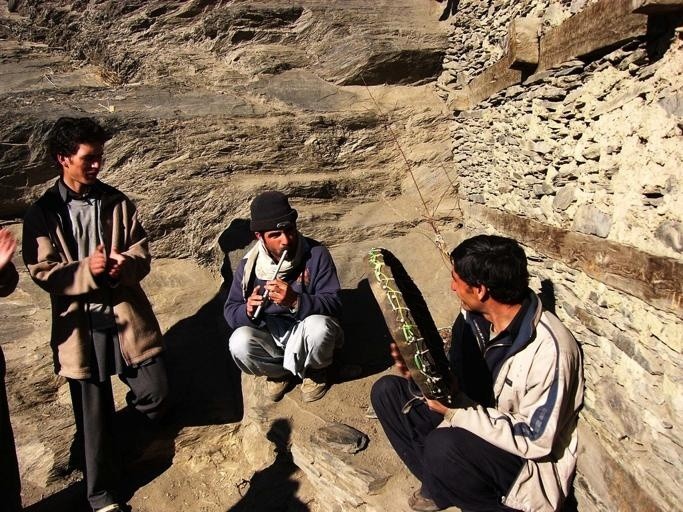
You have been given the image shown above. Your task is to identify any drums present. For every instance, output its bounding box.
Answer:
[363,248,454,409]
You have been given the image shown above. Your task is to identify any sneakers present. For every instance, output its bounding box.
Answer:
[301,377,328,402]
[408,488,444,511]
[264,380,289,401]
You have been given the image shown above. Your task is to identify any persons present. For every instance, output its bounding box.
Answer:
[223,192,345,403]
[0,223,23,512]
[367,235,584,512]
[23,117,170,512]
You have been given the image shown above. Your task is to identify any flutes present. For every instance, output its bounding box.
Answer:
[251,250,287,321]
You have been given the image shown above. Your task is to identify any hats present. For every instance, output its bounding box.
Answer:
[250,190,298,232]
